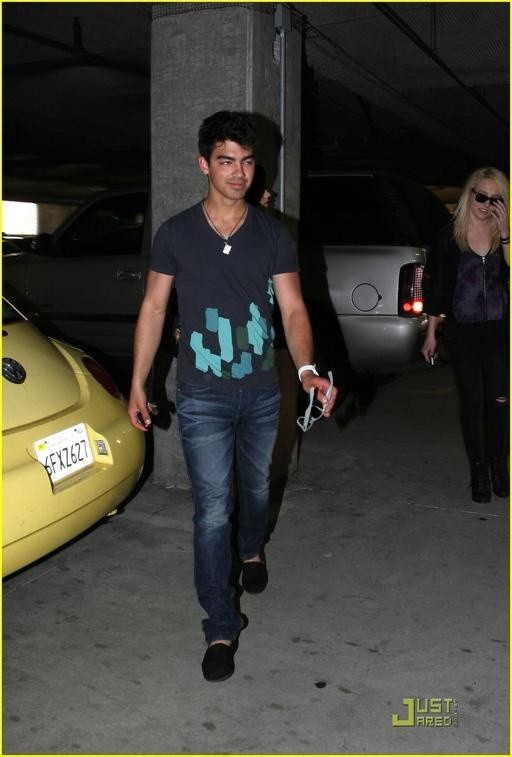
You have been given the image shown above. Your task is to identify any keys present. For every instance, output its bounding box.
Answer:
[431,356,435,366]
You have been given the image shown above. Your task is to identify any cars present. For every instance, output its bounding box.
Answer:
[1,295,147,582]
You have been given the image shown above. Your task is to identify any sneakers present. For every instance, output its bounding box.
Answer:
[471,461,491,503]
[491,459,509,498]
[201,634,241,681]
[241,549,270,595]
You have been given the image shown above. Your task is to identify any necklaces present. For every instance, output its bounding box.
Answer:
[202,197,250,257]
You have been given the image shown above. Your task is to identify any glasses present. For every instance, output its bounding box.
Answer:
[471,187,504,207]
[296,369,335,433]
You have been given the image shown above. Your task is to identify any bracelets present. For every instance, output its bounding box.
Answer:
[297,363,322,383]
[500,236,510,241]
[500,241,510,244]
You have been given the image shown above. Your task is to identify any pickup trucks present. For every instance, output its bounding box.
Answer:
[4,188,432,370]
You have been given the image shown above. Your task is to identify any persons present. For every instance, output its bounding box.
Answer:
[251,174,273,208]
[127,109,341,684]
[420,164,511,504]
